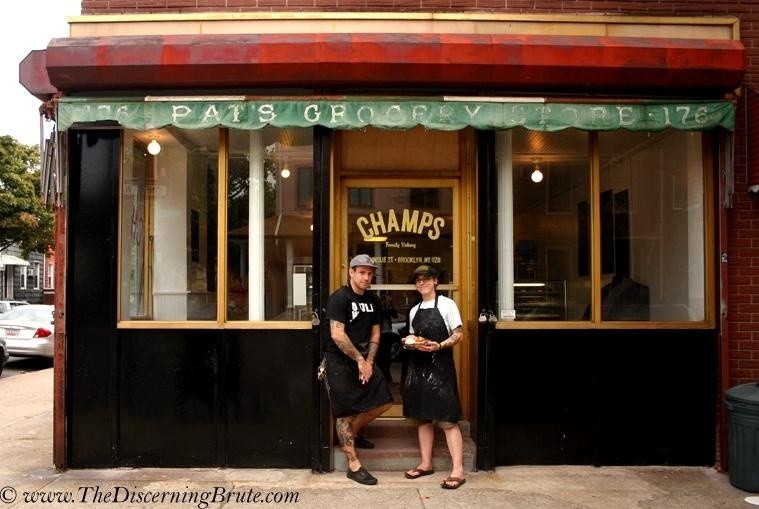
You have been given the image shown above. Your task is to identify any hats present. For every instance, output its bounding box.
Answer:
[350,255,379,270]
[410,265,437,277]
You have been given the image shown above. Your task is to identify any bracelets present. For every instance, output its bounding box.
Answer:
[437,343,442,350]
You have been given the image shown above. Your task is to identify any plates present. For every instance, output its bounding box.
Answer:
[401,344,427,350]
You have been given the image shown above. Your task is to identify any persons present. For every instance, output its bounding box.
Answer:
[318,253,396,488]
[400,264,467,490]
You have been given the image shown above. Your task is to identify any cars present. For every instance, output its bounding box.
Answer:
[0,336,10,379]
[0,300,31,314]
[0,303,55,359]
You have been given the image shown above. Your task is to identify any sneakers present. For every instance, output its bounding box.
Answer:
[346,467,378,485]
[354,435,375,449]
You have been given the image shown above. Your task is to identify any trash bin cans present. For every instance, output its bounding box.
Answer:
[725,382,759,493]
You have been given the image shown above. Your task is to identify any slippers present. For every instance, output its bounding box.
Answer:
[440,476,465,489]
[405,466,433,478]
